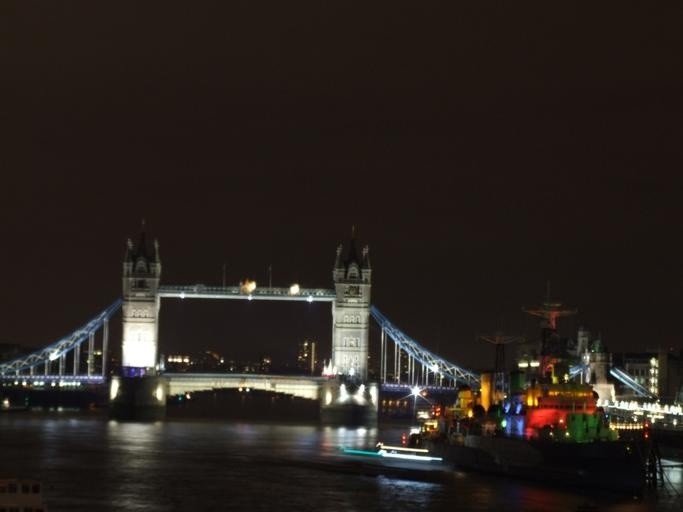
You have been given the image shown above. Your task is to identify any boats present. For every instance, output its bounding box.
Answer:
[0,476,46,512]
[337,439,449,476]
[405,273,659,502]
